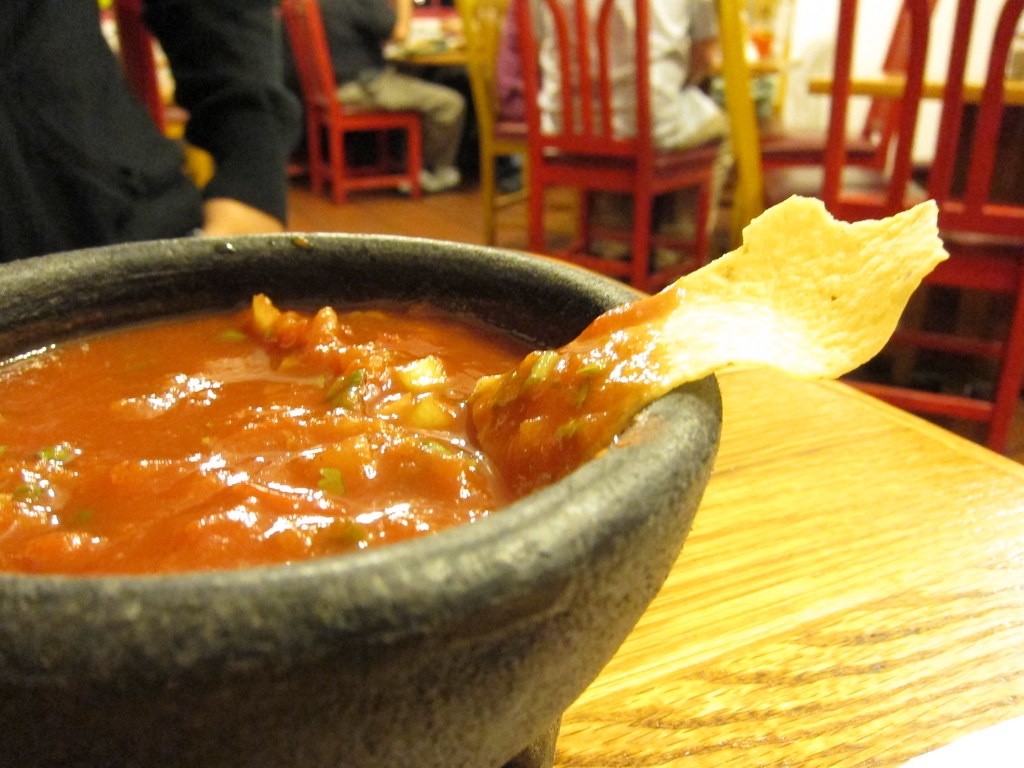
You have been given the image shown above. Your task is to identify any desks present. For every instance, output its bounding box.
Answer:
[808,77,1023,204]
[387,45,467,68]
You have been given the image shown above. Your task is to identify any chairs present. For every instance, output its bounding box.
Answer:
[283,0,423,203]
[451,0,1024,456]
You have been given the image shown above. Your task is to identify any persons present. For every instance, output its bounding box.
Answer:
[1,0,304,261]
[317,0,466,193]
[497,0,775,269]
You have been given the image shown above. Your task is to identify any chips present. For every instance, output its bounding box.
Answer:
[472,195,949,474]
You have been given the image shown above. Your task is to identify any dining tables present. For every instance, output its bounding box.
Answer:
[536,350,1024,768]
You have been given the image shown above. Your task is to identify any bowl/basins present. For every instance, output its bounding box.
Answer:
[2,230,724,765]
